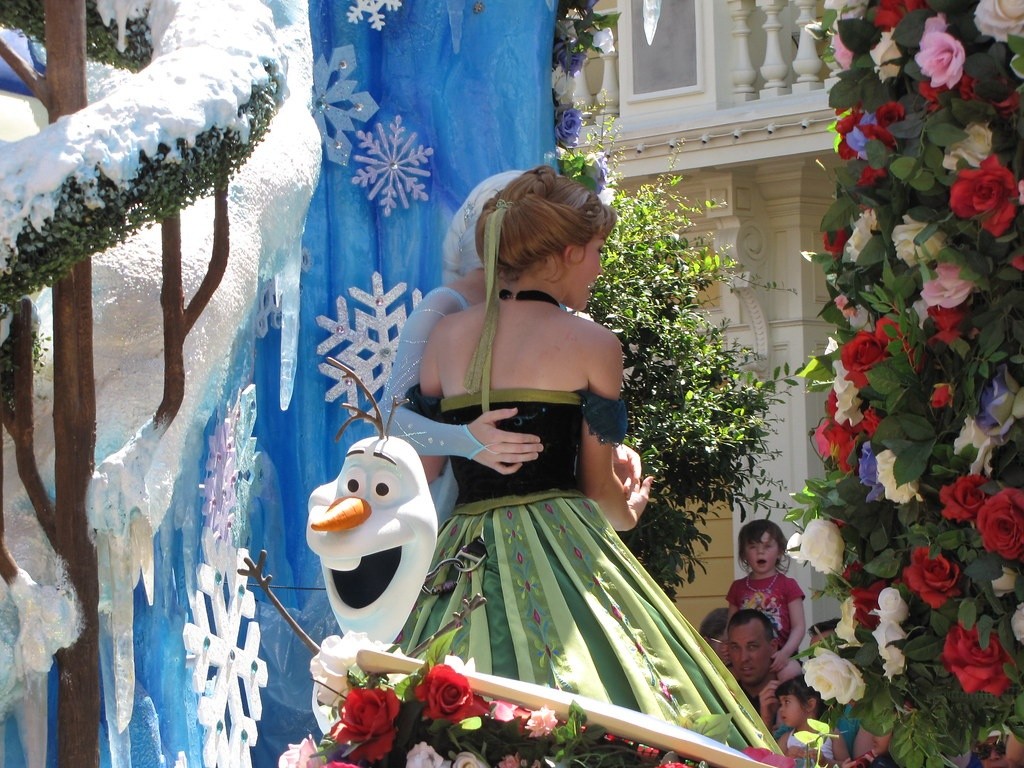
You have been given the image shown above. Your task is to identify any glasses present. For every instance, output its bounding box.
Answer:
[975,735,1009,760]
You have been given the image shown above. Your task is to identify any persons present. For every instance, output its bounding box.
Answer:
[378,163,785,756]
[720,519,806,683]
[699,609,1024,768]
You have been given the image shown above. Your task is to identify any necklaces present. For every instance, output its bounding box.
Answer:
[746,572,778,591]
[499,289,559,306]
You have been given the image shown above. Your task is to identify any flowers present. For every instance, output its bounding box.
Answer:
[276,0,1024,768]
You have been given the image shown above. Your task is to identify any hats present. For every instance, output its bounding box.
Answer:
[987,724,1013,736]
